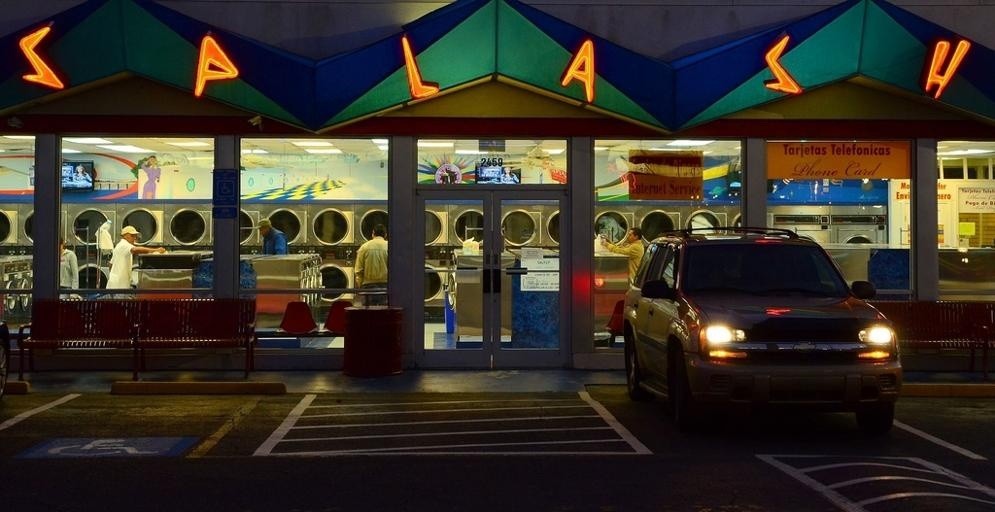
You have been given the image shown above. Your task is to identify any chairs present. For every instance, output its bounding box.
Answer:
[323,300,354,335]
[606,300,624,347]
[868,301,995,377]
[280,302,317,335]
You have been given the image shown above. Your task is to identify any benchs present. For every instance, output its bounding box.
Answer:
[18,297,255,380]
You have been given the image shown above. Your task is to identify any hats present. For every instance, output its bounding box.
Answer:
[121,226,139,235]
[254,219,270,229]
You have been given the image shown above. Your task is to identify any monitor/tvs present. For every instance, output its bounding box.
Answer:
[727,171,773,197]
[61,160,94,192]
[475,162,521,185]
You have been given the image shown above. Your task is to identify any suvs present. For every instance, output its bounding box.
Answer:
[623,225,905,439]
[594,252,633,316]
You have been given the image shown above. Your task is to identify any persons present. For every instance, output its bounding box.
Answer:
[105,225,166,299]
[255,218,289,255]
[354,224,388,305]
[500,167,519,184]
[72,166,92,188]
[600,228,646,291]
[59,237,82,302]
[140,156,162,199]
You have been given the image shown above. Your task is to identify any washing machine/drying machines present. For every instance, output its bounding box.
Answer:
[1,201,889,323]
